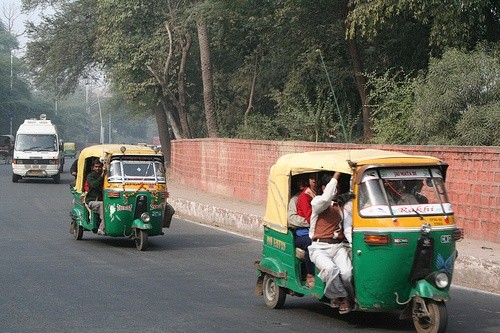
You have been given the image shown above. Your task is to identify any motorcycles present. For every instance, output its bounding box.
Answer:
[68,142,170,251]
[252,147,464,333]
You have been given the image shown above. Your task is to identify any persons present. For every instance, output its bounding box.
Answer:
[289,174,315,289]
[343,175,369,260]
[308,172,354,314]
[362,180,419,210]
[403,180,428,204]
[70,160,78,179]
[84,181,91,220]
[87,160,108,235]
[297,173,318,224]
[161,203,175,235]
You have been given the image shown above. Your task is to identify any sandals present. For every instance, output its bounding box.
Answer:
[306,274,315,287]
[339,298,351,314]
[331,298,342,307]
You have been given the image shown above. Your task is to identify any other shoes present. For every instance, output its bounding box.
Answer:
[97,229,106,234]
[162,231,164,235]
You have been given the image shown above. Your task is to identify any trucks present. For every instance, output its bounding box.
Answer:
[0,114,77,185]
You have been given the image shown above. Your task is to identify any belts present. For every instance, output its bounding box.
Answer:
[313,238,342,244]
